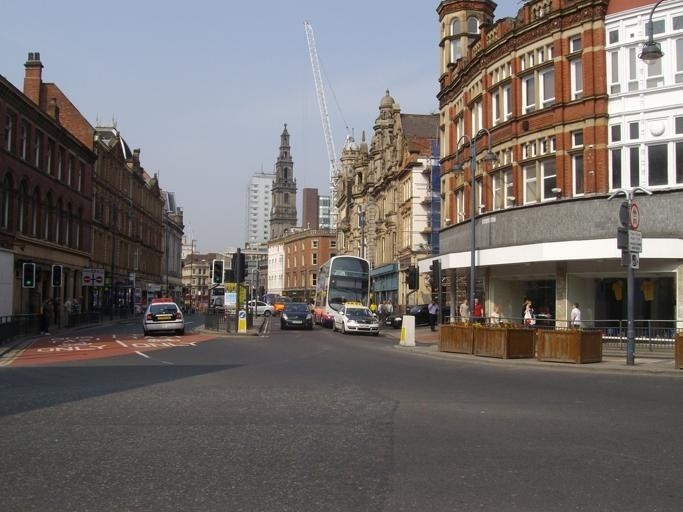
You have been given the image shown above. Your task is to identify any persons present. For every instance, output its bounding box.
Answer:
[491,305,507,323]
[40,294,83,336]
[460,299,470,324]
[524,301,536,328]
[570,303,581,329]
[428,300,440,331]
[473,297,484,323]
[368,300,394,324]
[521,296,530,324]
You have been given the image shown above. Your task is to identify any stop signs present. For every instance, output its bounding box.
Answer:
[96,277,103,283]
[84,276,90,283]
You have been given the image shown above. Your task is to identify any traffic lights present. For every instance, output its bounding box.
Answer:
[22,263,36,288]
[212,259,224,284]
[52,265,62,287]
[405,264,419,290]
[243,261,249,276]
[428,260,439,289]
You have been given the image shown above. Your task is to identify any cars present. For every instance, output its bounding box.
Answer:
[386,303,450,329]
[143,297,185,336]
[332,301,381,337]
[246,293,313,330]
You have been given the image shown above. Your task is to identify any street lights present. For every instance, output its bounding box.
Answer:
[189,239,198,315]
[450,126,498,327]
[360,203,367,260]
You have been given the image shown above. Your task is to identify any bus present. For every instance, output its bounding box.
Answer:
[313,255,374,330]
[209,285,224,312]
